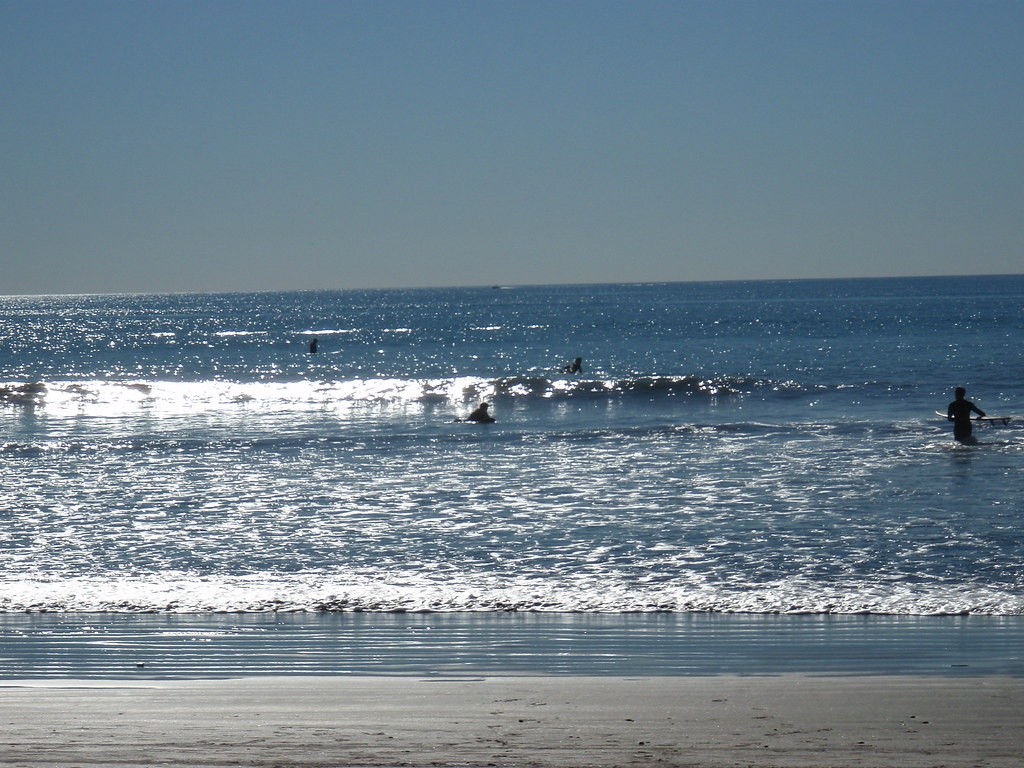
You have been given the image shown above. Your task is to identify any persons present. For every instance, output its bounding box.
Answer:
[561,357,583,374]
[947,387,986,442]
[454,402,496,423]
[310,338,318,353]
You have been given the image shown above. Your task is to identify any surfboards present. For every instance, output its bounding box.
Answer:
[934,411,1012,427]
